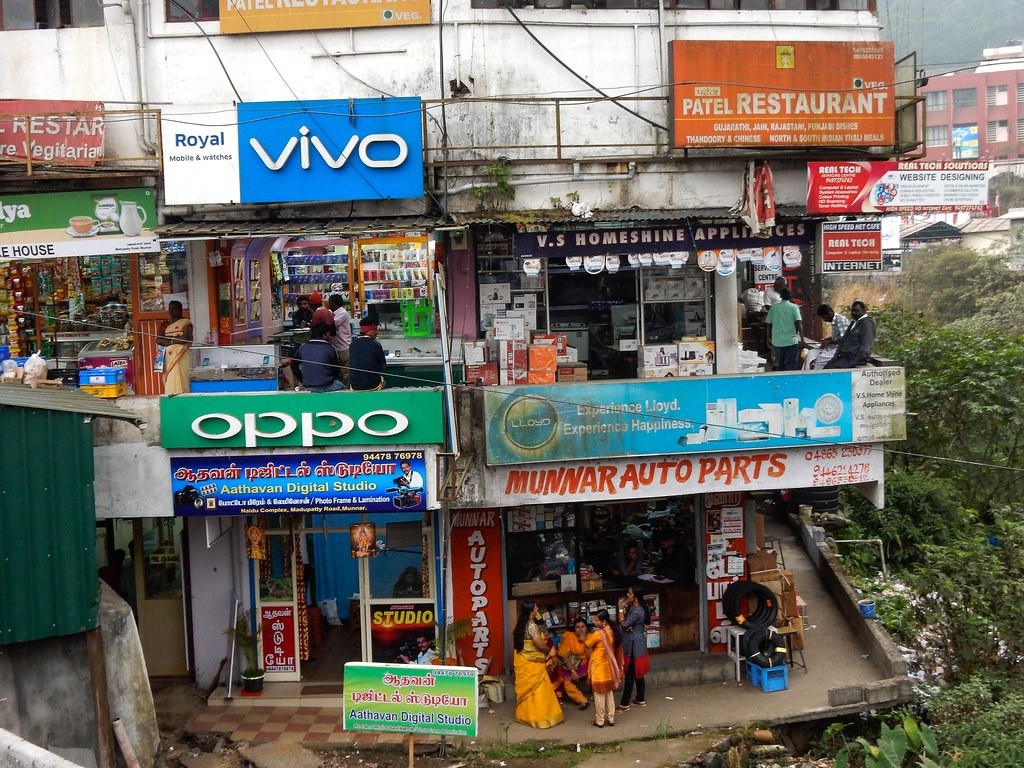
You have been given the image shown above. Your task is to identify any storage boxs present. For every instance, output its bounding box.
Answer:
[747,514,808,649]
[535,573,659,651]
[464,243,707,388]
[0,346,127,398]
[746,660,788,692]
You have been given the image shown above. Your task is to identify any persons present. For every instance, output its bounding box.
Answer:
[99,540,154,617]
[613,537,693,587]
[802,301,876,370]
[156,301,193,393]
[290,322,346,391]
[400,462,423,493]
[349,317,386,392]
[515,586,651,729]
[739,277,805,372]
[396,567,422,594]
[402,635,434,664]
[293,294,351,385]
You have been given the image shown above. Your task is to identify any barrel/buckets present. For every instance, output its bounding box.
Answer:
[859,599,876,619]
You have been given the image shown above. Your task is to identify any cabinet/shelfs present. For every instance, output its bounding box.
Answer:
[531,576,699,655]
[478,233,712,347]
[281,237,430,322]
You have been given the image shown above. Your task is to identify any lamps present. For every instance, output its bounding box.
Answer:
[916,69,929,88]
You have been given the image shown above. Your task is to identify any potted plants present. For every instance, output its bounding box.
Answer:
[220,615,266,692]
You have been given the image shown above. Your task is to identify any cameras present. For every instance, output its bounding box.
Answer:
[393,476,407,485]
[175,485,204,509]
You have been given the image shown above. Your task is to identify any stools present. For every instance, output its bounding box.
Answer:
[764,536,785,570]
[308,607,324,640]
[748,311,768,355]
[777,626,807,674]
[400,299,432,337]
[349,601,360,633]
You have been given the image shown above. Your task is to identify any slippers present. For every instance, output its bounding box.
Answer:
[614,705,630,715]
[631,698,646,706]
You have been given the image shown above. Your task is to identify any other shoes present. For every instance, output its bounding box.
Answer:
[607,720,614,726]
[593,718,604,728]
[579,701,589,709]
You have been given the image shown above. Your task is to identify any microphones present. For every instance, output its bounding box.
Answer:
[386,488,399,492]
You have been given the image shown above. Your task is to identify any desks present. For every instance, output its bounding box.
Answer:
[727,625,778,681]
[268,330,310,358]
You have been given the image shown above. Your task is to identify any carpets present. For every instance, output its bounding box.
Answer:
[301,684,343,695]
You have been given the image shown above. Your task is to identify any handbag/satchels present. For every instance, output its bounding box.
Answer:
[153,345,165,373]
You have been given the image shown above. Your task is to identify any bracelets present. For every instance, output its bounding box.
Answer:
[618,609,624,613]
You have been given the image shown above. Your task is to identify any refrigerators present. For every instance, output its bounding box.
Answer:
[78,342,136,396]
